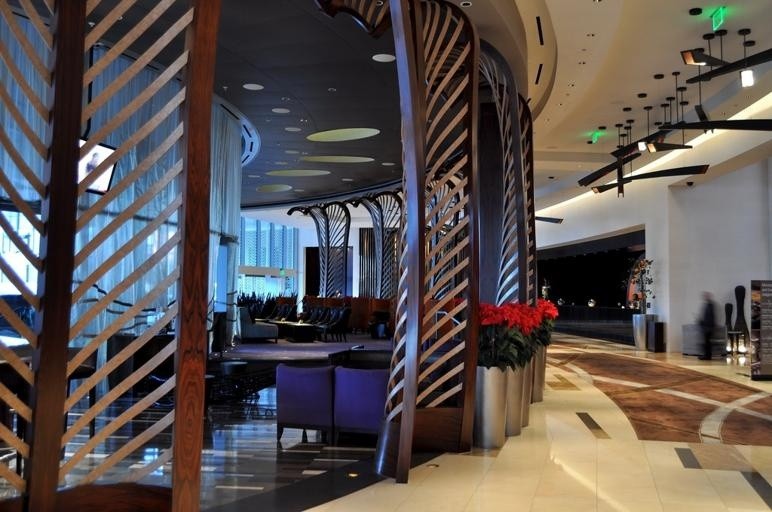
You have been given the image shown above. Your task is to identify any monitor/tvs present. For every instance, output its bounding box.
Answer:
[77,136,118,195]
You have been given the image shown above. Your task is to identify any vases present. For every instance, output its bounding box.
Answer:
[476,341,549,453]
[632,314,658,349]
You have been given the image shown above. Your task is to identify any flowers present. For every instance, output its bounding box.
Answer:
[474,293,560,370]
[624,257,656,311]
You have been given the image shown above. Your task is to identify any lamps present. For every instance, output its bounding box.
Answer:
[579,23,772,199]
